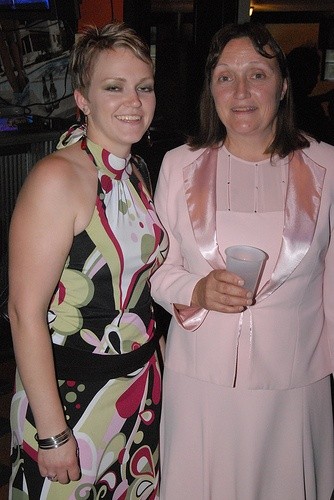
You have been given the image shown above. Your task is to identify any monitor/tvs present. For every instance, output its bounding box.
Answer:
[0,0,84,147]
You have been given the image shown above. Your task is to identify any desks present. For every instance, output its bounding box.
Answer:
[0,119,77,324]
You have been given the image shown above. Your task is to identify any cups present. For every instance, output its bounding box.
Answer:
[224,245,266,298]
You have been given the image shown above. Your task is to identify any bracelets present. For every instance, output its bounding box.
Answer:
[34,427,74,450]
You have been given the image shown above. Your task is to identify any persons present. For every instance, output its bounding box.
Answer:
[8,20,174,499]
[150,22,333,500]
